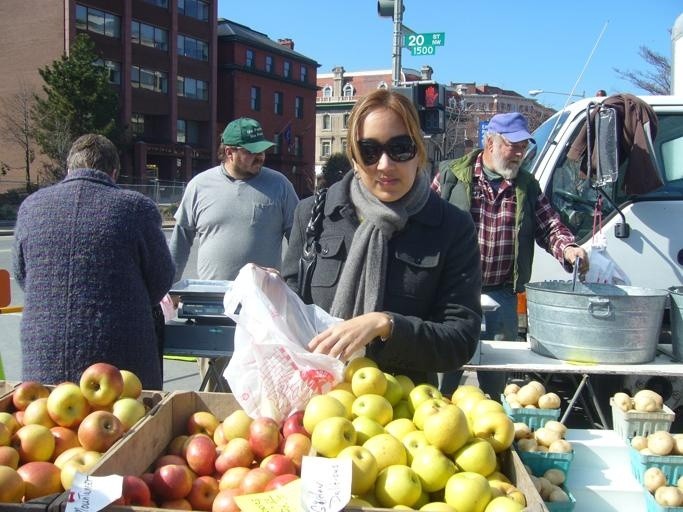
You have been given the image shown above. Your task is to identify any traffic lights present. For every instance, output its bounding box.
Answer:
[377,0,394,19]
[413,82,444,133]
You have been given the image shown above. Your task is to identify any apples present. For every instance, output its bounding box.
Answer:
[303,355,527,512]
[115,408,311,512]
[0,362,151,505]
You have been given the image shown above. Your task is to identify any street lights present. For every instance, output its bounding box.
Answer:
[527,90,586,99]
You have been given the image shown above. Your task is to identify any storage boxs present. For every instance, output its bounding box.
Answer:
[0,379,554,512]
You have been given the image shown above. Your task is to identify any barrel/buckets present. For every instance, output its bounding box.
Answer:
[668,286,683,361]
[523,280,670,365]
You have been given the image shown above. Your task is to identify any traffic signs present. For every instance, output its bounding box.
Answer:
[404,32,445,55]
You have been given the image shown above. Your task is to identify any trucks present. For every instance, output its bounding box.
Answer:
[514,13,682,342]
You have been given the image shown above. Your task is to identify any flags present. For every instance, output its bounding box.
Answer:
[283,123,291,146]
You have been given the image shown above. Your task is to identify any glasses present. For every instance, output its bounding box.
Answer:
[351,134,417,166]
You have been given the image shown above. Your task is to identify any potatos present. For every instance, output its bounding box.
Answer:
[505,379,683,507]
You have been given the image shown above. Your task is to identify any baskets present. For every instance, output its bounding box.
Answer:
[609,397,675,446]
[629,445,683,512]
[500,394,576,512]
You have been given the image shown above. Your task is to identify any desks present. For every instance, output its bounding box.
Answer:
[158,314,234,392]
[479,291,500,315]
[454,340,682,432]
[565,427,653,512]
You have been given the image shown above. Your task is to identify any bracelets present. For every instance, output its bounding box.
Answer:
[380,318,395,341]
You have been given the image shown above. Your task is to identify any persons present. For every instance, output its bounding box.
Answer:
[279,90,481,389]
[9,133,175,390]
[430,113,588,342]
[170,116,299,286]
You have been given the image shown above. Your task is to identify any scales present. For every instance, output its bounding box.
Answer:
[168,279,242,324]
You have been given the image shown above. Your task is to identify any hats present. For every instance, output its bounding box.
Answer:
[221,118,280,154]
[487,112,536,144]
